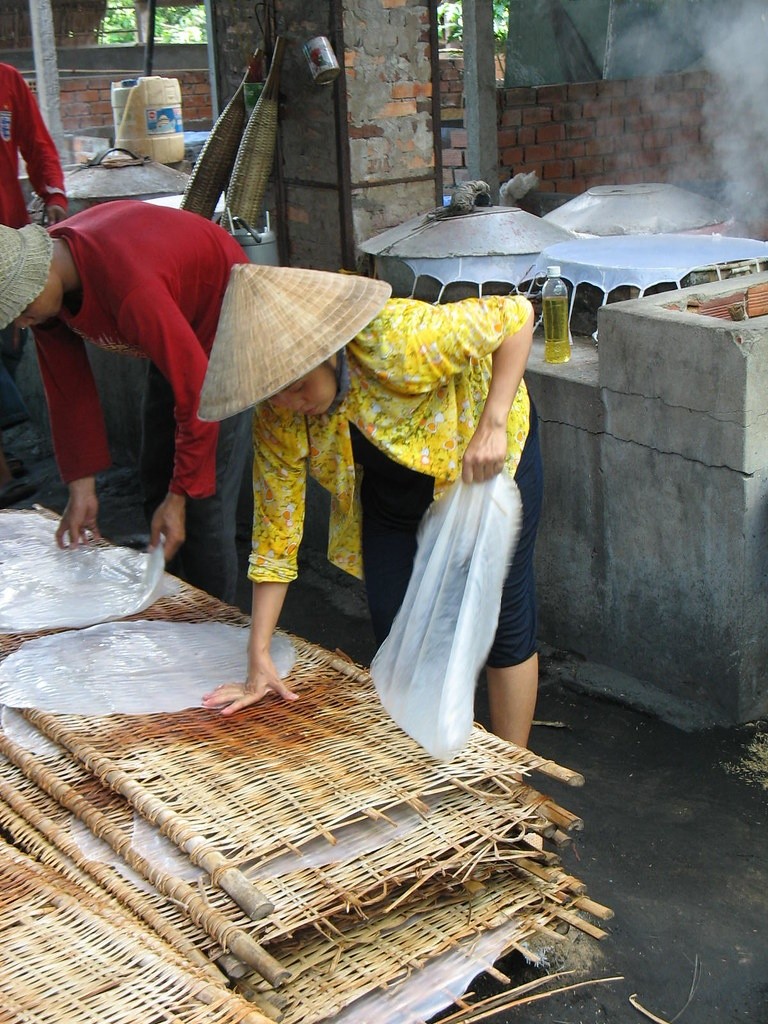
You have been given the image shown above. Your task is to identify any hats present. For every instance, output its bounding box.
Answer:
[0,223,54,330]
[197,263,392,422]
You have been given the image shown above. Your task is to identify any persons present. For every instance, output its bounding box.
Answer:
[0,59,67,403]
[0,200,255,604]
[194,261,543,752]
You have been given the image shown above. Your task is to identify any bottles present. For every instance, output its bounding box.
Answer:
[541,266,572,364]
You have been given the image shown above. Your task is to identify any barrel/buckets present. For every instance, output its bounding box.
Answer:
[114,76,184,163]
[225,216,280,267]
[111,79,138,142]
[304,35,342,85]
